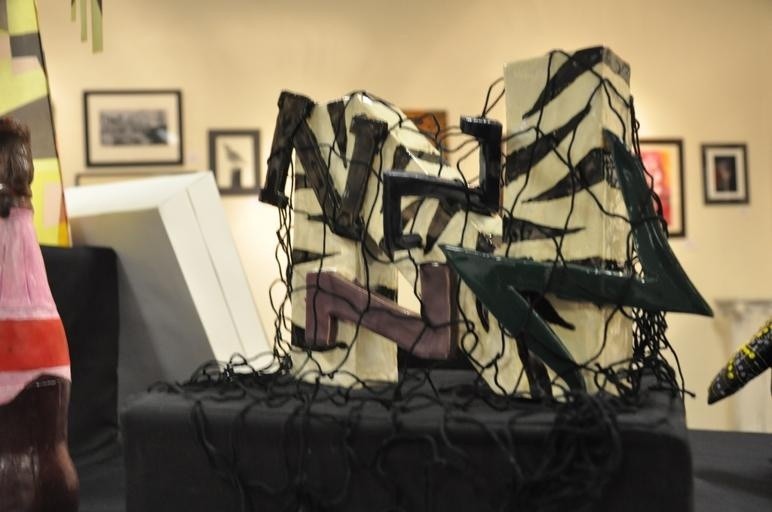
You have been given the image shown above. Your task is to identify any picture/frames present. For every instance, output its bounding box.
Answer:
[83,89,184,166]
[208,129,262,194]
[636,137,685,237]
[401,108,449,167]
[701,141,750,206]
